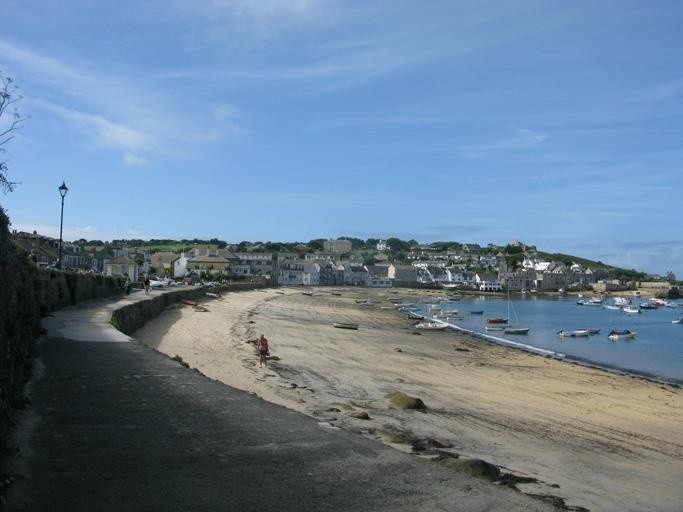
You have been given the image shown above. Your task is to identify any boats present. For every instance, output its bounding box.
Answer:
[606,326,639,345]
[556,327,587,339]
[180,299,199,306]
[301,290,322,296]
[583,329,600,334]
[574,289,683,326]
[205,292,220,299]
[328,290,533,335]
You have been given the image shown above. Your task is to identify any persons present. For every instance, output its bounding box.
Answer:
[29,249,37,267]
[125,277,132,295]
[143,276,150,295]
[55,257,61,269]
[201,278,204,287]
[258,334,269,369]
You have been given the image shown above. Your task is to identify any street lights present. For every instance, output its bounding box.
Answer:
[56,180,70,271]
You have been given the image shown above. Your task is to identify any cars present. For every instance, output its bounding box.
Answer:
[43,262,107,276]
[143,273,221,288]
